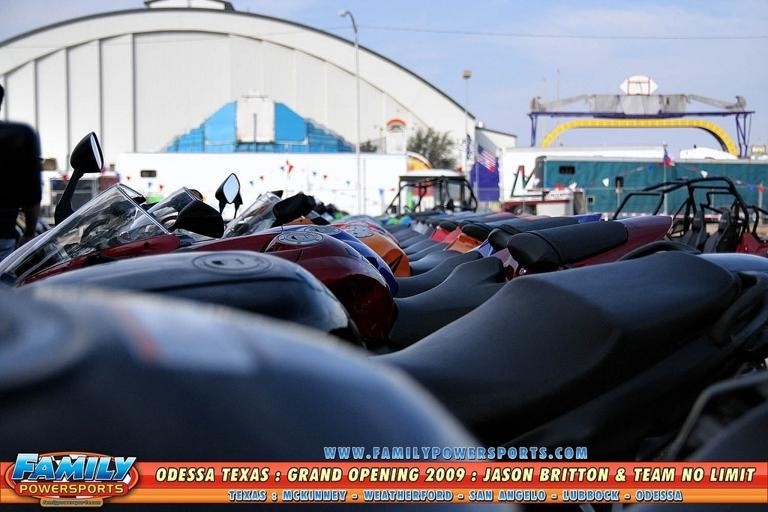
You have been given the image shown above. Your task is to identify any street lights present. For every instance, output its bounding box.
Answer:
[340,10,363,210]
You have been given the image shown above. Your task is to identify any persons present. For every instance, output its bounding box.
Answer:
[0,84,43,259]
